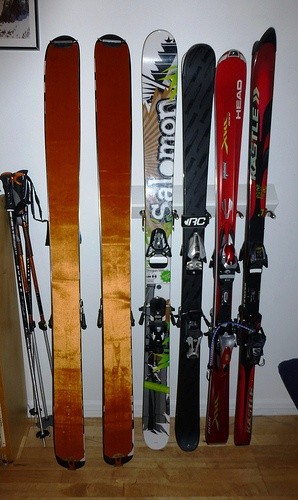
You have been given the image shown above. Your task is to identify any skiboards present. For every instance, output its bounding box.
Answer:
[233,26,278,447]
[208,49,249,446]
[174,42,212,452]
[43,34,133,472]
[138,29,176,451]
